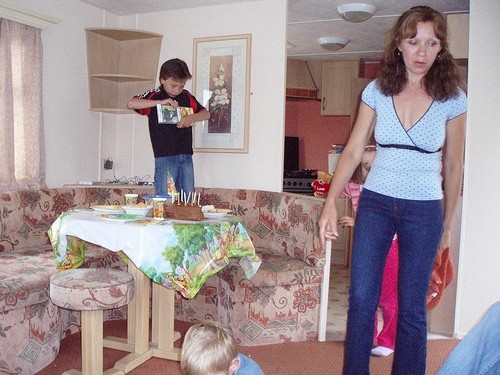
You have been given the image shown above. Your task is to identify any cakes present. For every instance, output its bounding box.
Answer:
[164,200,204,220]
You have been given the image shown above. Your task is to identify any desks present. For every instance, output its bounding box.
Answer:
[48,206,262,374]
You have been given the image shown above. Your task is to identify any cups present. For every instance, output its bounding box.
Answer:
[125,193,138,205]
[153,197,166,220]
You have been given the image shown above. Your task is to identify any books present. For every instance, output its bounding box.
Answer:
[157,104,196,126]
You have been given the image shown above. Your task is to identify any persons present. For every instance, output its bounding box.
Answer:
[180,320,265,375]
[318,6,469,375]
[435,301,500,375]
[337,163,399,356]
[128,58,210,202]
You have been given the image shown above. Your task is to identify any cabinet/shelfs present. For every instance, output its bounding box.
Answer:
[330,197,350,266]
[320,62,361,116]
[85,26,164,114]
[446,10,470,59]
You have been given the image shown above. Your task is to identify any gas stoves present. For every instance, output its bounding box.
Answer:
[283,170,317,190]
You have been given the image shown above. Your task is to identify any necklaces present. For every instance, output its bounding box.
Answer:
[408,85,425,94]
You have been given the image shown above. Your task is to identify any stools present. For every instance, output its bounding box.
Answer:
[50,267,134,375]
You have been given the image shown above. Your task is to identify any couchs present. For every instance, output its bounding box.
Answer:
[1,186,333,374]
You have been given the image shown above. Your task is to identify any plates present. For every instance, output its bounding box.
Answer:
[203,212,227,219]
[90,205,125,214]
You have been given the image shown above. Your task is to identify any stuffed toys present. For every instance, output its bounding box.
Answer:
[361,151,376,169]
[311,171,332,198]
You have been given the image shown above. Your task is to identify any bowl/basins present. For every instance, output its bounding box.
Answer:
[121,205,153,216]
[145,195,172,207]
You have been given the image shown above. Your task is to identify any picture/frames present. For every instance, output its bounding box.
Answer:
[190,33,251,154]
